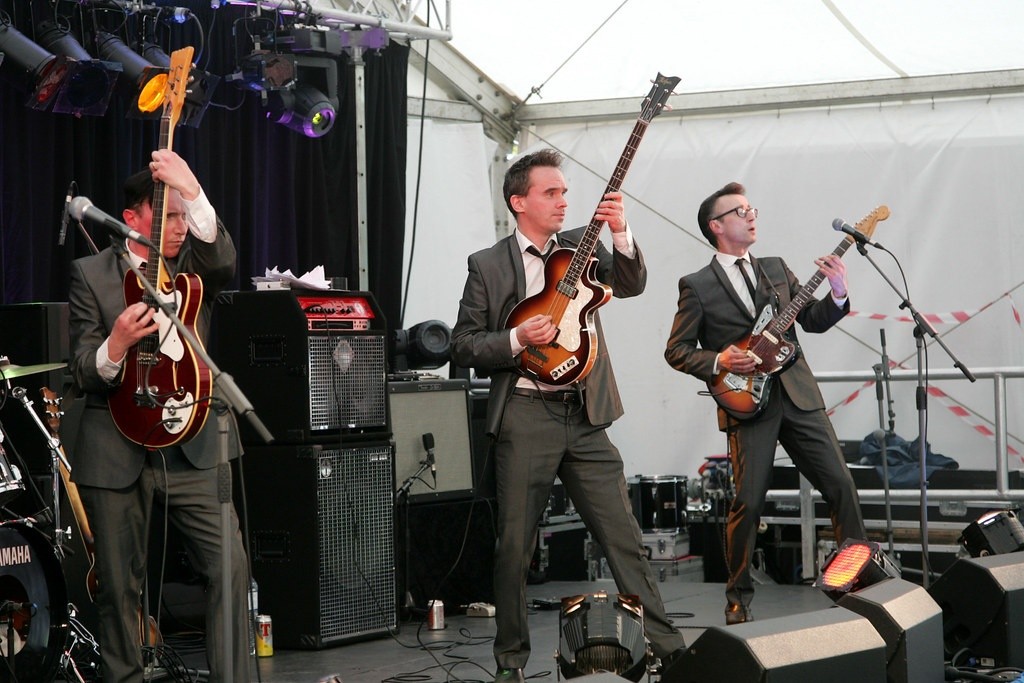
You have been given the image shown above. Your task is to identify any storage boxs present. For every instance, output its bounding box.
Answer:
[534,477,605,582]
[601,473,803,583]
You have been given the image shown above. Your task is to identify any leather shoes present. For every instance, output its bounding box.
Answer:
[725,603,754,623]
[659,645,688,671]
[494,663,524,683]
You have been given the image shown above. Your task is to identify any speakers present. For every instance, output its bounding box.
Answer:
[833,574,944,682]
[0,302,95,637]
[386,379,475,502]
[211,289,391,445]
[224,440,401,653]
[927,552,1024,673]
[659,604,888,683]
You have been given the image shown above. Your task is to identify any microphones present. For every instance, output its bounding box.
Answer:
[422,433,436,479]
[832,218,884,249]
[58,195,72,245]
[68,195,152,248]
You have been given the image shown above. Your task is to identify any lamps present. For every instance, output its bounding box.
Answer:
[813,538,902,605]
[554,589,655,683]
[0,0,339,137]
[957,510,1024,558]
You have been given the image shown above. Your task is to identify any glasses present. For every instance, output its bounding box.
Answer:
[713,206,758,219]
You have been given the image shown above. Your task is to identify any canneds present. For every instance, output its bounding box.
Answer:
[255,614,273,656]
[427,600,445,629]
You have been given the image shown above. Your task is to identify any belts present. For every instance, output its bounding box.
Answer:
[514,387,578,405]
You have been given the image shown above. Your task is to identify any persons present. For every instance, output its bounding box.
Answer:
[452,148,691,683]
[664,183,870,624]
[69,150,259,683]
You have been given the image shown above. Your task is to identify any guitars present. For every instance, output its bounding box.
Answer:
[501,72,680,387]
[104,45,216,453]
[36,382,163,655]
[704,205,890,426]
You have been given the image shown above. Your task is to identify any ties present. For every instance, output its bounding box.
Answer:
[526,238,556,263]
[734,258,756,305]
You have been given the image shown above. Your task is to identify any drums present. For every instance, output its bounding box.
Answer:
[623,473,690,533]
[0,437,27,497]
[0,519,70,683]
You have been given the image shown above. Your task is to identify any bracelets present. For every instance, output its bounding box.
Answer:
[836,292,848,300]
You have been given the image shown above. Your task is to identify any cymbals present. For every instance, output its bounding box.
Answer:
[0,362,69,381]
